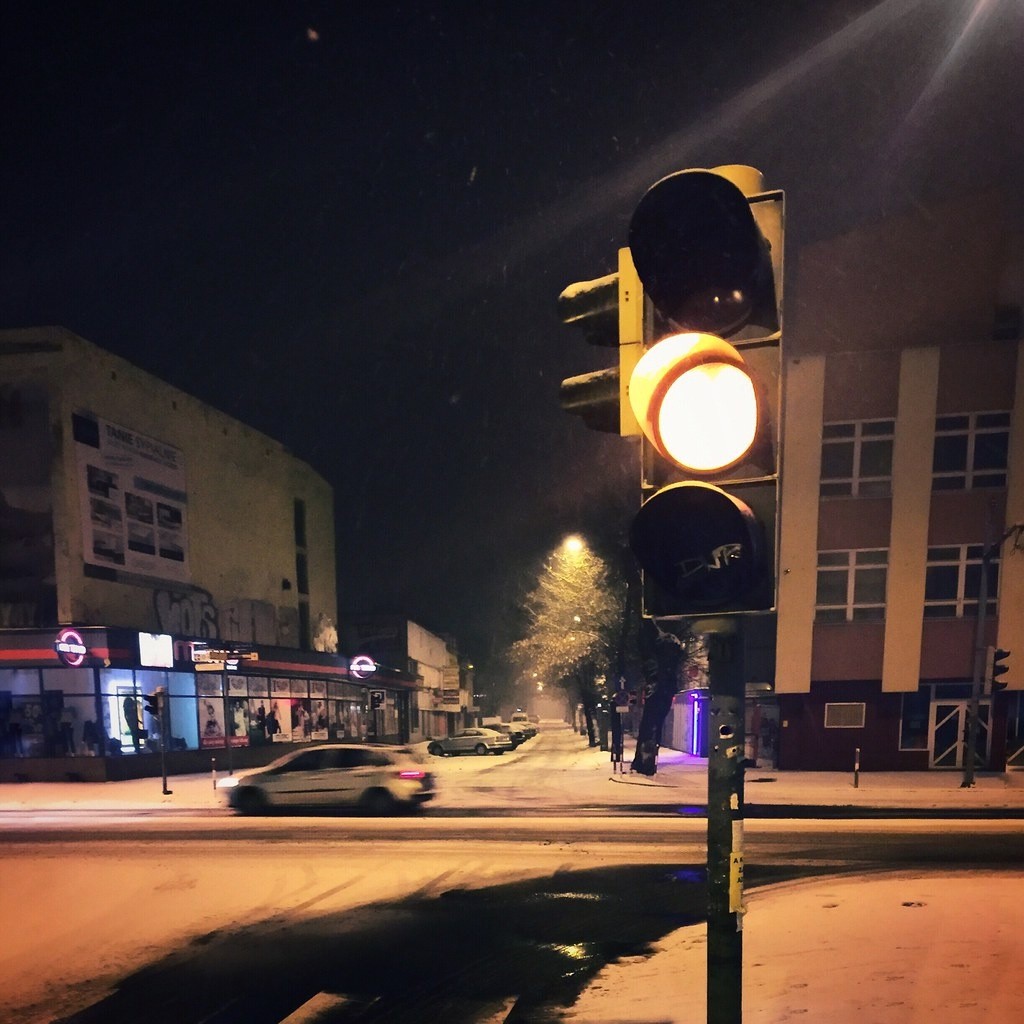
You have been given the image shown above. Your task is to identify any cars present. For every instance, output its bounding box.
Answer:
[216,745,438,818]
[479,712,541,744]
[427,728,513,756]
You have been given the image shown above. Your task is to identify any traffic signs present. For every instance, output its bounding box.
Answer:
[615,676,629,713]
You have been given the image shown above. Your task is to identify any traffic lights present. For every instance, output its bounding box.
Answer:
[615,163,782,619]
